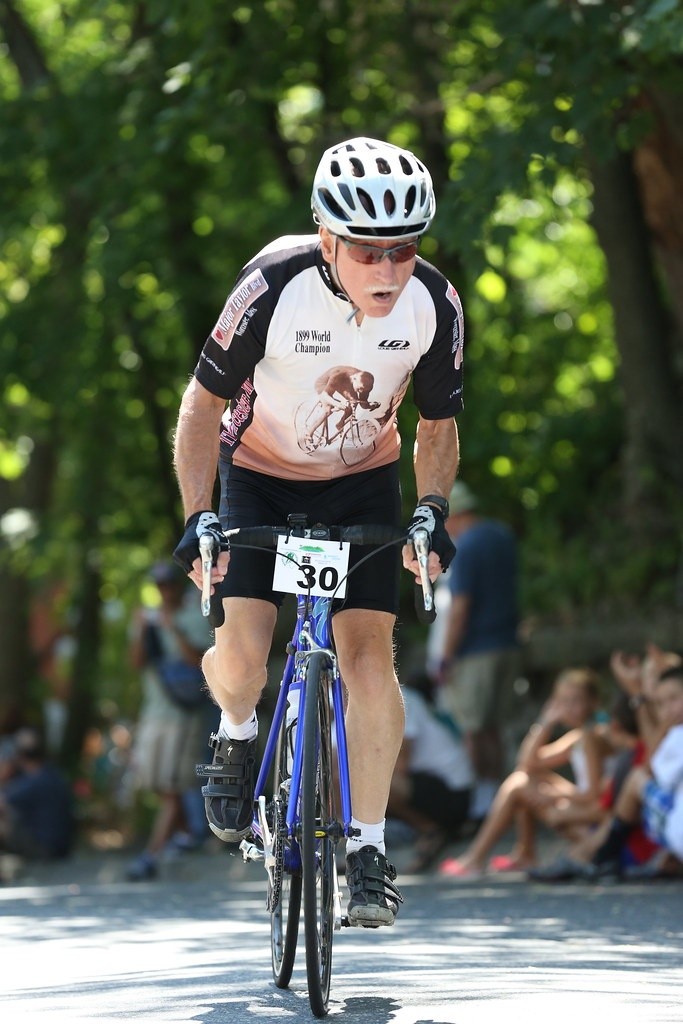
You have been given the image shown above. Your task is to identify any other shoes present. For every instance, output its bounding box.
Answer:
[124,855,161,881]
[436,857,482,884]
[484,857,527,884]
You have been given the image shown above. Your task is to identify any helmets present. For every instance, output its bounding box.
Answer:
[310,136,435,240]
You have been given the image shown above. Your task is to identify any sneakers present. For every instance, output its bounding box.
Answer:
[527,853,604,889]
[196,731,259,843]
[343,845,404,927]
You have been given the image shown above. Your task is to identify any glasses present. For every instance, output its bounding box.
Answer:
[336,234,421,264]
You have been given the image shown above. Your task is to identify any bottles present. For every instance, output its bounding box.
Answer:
[287,682,302,775]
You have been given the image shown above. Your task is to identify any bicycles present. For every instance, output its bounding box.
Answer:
[191,504,439,1022]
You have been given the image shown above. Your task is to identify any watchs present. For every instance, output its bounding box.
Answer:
[417,494,450,520]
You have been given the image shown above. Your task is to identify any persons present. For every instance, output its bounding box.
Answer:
[430,642,683,887]
[0,549,229,886]
[429,480,535,812]
[325,627,474,875]
[170,136,466,927]
